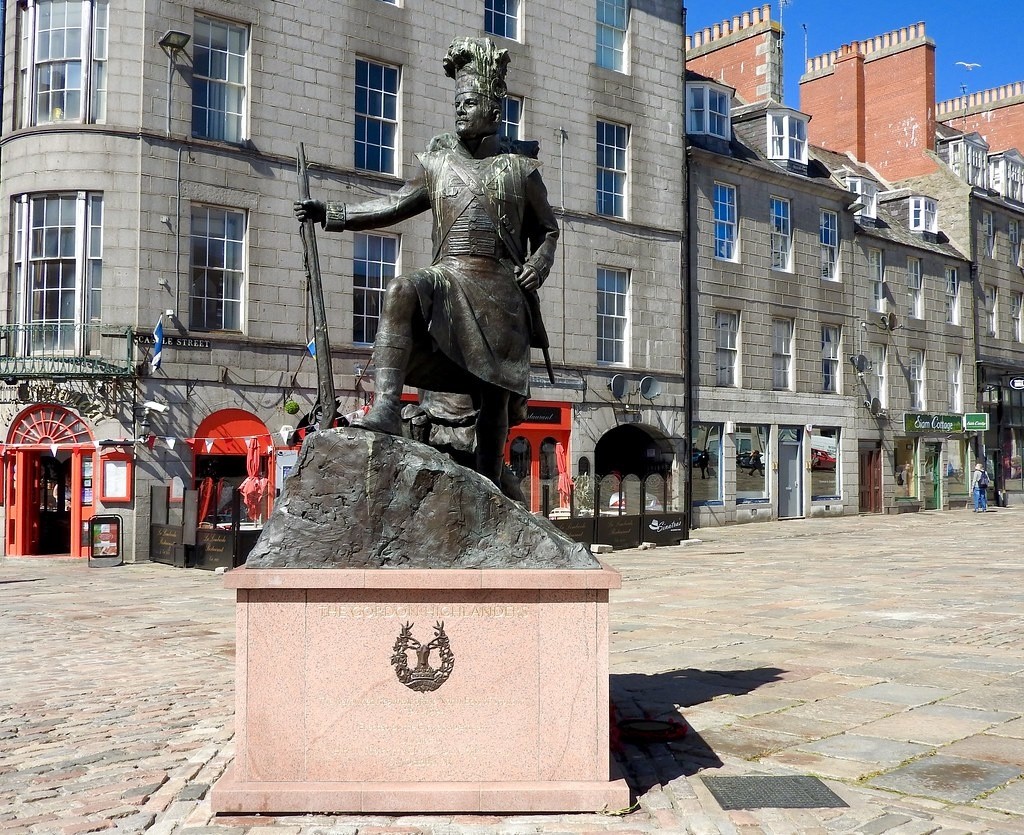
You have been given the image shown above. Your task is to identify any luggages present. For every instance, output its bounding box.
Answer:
[707,464,717,478]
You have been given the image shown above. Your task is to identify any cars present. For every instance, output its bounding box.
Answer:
[737,450,765,469]
[811,449,837,471]
[693,452,718,467]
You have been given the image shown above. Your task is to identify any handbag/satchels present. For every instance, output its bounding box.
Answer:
[749,456,755,464]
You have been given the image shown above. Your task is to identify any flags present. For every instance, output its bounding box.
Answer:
[151,313,164,374]
[306,338,317,361]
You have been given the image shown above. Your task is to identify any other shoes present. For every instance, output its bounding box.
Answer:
[747,473,754,477]
[701,476,705,479]
[973,510,978,512]
[981,509,986,512]
[760,475,765,478]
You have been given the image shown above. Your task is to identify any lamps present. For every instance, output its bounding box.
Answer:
[159,30,191,49]
[138,414,151,445]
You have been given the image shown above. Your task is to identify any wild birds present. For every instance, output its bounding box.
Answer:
[955,61,981,71]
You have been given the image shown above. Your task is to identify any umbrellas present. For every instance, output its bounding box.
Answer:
[238,436,276,529]
[554,442,571,511]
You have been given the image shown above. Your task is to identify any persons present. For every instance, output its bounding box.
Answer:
[900,463,913,497]
[736,449,764,478]
[292,36,559,492]
[693,442,710,479]
[972,463,991,513]
[53,484,71,511]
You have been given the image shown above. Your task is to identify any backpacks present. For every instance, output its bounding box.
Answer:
[897,471,904,485]
[975,469,989,488]
